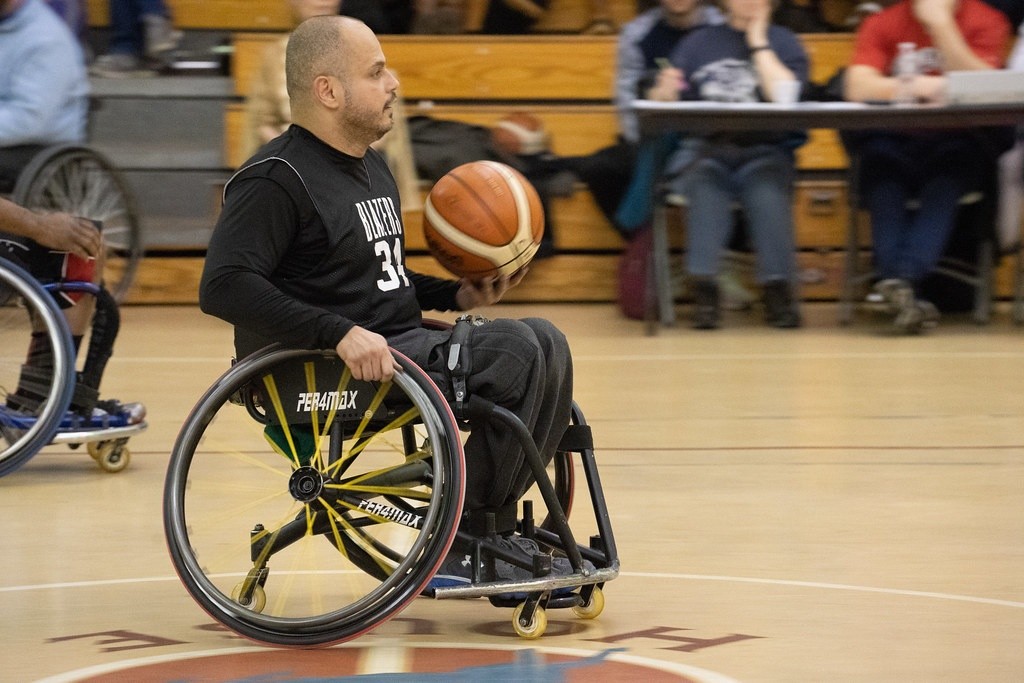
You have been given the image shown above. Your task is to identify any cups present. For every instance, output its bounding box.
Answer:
[775,80,800,103]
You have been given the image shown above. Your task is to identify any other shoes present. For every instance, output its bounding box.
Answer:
[97,397,148,424]
[758,280,800,327]
[686,280,720,328]
[894,298,940,328]
[140,14,184,58]
[89,51,168,77]
[867,276,912,312]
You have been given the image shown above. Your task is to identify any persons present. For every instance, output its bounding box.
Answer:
[87,1,174,83]
[197,15,604,605]
[613,0,756,312]
[641,0,808,325]
[1,194,146,452]
[0,0,89,308]
[841,0,1013,333]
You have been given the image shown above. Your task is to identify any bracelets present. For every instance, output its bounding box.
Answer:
[748,45,773,54]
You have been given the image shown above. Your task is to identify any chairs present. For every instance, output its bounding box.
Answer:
[839,187,993,325]
[654,192,749,327]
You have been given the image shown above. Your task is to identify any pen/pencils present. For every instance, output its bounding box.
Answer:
[654,55,692,95]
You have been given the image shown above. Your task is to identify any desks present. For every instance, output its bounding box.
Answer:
[627,97,1024,335]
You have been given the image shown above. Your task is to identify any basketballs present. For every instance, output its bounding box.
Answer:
[424,159,545,283]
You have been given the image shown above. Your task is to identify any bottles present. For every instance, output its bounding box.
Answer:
[893,42,922,107]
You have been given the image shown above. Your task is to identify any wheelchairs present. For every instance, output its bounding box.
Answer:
[11,144,139,309]
[0,255,149,479]
[163,323,619,650]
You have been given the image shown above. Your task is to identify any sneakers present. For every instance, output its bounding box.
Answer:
[421,532,596,600]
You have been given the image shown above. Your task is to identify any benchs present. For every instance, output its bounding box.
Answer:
[226,26,1024,303]
